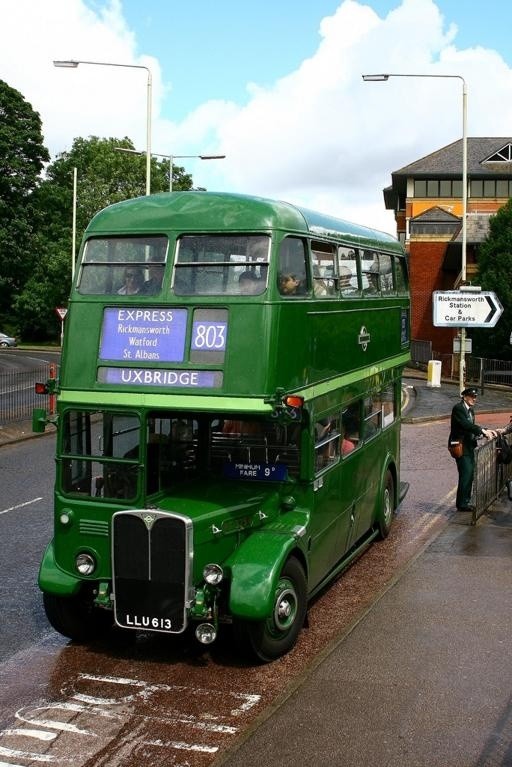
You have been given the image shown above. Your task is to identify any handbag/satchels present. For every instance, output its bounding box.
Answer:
[449,442,463,459]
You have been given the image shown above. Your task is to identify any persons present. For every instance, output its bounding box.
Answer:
[495,423,512,434]
[238,271,258,296]
[116,265,144,294]
[139,255,191,295]
[169,419,188,438]
[256,255,267,292]
[279,267,300,294]
[302,259,392,297]
[331,417,354,454]
[447,387,497,513]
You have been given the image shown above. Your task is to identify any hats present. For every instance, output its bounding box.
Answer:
[461,388,479,396]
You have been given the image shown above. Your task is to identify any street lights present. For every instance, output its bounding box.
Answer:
[115,148,225,192]
[53,59,151,286]
[360,73,469,405]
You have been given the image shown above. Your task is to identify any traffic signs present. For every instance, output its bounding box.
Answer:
[433,290,503,329]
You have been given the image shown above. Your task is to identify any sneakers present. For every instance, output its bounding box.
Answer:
[457,505,475,512]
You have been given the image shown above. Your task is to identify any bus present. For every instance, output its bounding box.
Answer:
[32,192,413,664]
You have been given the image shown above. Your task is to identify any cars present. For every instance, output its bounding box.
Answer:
[0,332,17,349]
